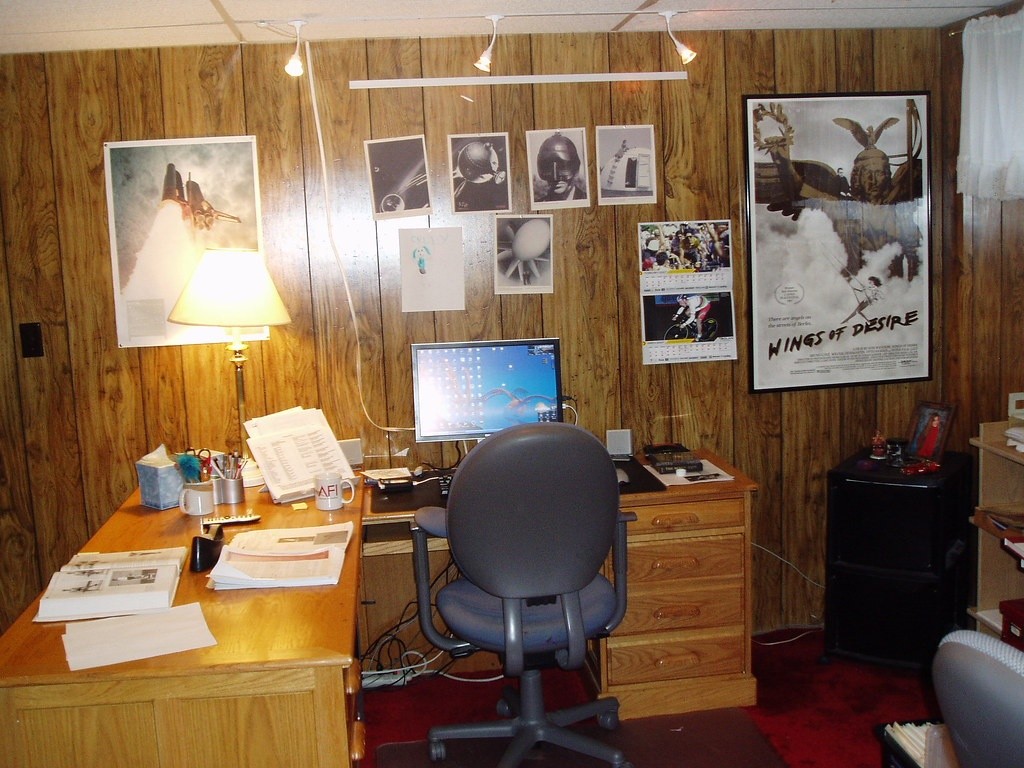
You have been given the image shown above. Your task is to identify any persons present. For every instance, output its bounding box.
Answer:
[537,131,587,201]
[112,574,150,581]
[614,139,628,161]
[672,294,710,341]
[62,584,97,592]
[916,413,940,459]
[67,570,99,577]
[641,223,730,273]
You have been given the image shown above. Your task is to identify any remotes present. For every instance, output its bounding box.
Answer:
[202,514,261,528]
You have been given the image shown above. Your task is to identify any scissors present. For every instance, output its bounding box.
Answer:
[186,446,210,462]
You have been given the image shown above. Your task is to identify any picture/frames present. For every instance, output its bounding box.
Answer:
[92,132,274,350]
[738,90,936,397]
[902,399,960,465]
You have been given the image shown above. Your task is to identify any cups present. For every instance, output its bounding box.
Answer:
[315,472,356,511]
[178,479,215,516]
[222,477,244,504]
[210,475,222,505]
[885,437,910,467]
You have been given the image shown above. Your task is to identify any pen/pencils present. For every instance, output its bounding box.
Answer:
[210,453,248,479]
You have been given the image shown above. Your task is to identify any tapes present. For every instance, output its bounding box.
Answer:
[201,524,219,539]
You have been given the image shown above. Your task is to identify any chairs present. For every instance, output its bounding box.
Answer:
[408,419,637,768]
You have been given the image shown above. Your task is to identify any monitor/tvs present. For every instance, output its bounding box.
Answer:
[410,337,564,443]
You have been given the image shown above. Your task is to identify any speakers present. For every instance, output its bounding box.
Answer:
[606,429,633,461]
[338,438,363,470]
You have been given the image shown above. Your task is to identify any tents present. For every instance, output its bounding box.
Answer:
[600,147,653,192]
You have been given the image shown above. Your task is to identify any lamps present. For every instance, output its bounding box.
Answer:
[163,244,296,489]
[284,19,308,77]
[659,9,697,64]
[473,14,505,71]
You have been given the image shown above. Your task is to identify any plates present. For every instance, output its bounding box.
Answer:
[869,454,886,460]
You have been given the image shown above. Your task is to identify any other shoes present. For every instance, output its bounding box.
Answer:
[695,334,702,341]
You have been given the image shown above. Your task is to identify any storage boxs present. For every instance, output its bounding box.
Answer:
[134,448,228,512]
[998,597,1024,653]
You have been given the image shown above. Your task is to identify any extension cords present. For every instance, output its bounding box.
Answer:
[361,669,411,688]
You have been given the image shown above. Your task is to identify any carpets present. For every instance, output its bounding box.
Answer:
[371,702,795,768]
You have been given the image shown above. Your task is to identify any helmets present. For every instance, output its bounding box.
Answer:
[676,294,687,302]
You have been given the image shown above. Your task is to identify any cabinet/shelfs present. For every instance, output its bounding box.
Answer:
[362,444,766,726]
[818,437,976,682]
[959,422,1024,652]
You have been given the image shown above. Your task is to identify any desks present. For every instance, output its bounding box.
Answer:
[0,463,369,768]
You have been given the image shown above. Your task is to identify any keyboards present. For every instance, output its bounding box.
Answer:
[438,472,454,496]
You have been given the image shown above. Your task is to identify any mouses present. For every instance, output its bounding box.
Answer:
[616,468,629,485]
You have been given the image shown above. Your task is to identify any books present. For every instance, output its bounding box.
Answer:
[246,438,282,504]
[31,546,188,622]
